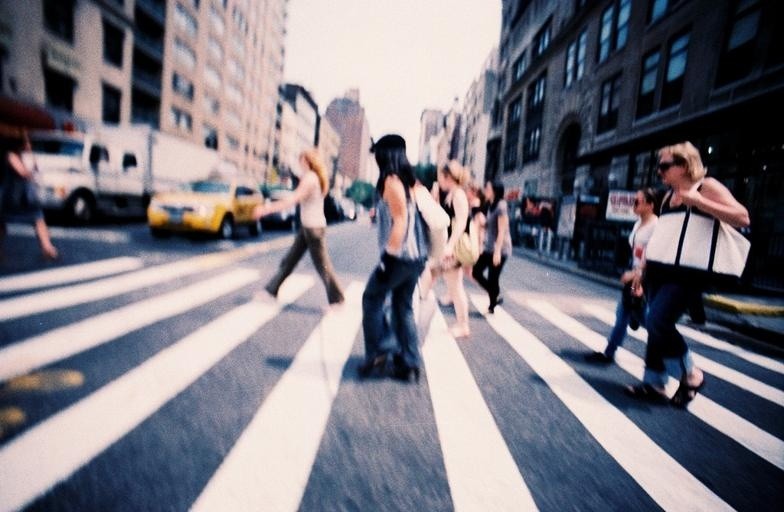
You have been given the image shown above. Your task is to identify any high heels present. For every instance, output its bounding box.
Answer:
[397,363,421,382]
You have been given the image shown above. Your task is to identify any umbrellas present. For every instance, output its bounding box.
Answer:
[1,95,58,128]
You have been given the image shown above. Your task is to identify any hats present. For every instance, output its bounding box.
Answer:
[369,134,407,151]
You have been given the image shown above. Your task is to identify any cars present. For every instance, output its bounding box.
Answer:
[261,183,377,233]
[146,163,270,240]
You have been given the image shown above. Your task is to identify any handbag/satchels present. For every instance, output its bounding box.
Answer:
[457,224,479,267]
[645,208,751,294]
[623,279,646,331]
[412,184,451,231]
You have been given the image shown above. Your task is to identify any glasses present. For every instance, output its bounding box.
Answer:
[658,162,677,173]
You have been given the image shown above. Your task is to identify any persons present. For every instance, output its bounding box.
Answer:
[248,134,515,384]
[587,142,750,411]
[1,120,60,259]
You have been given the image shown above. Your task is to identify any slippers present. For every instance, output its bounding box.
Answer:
[669,378,703,407]
[625,382,669,404]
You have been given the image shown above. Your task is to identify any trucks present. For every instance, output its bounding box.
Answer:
[25,121,238,227]
